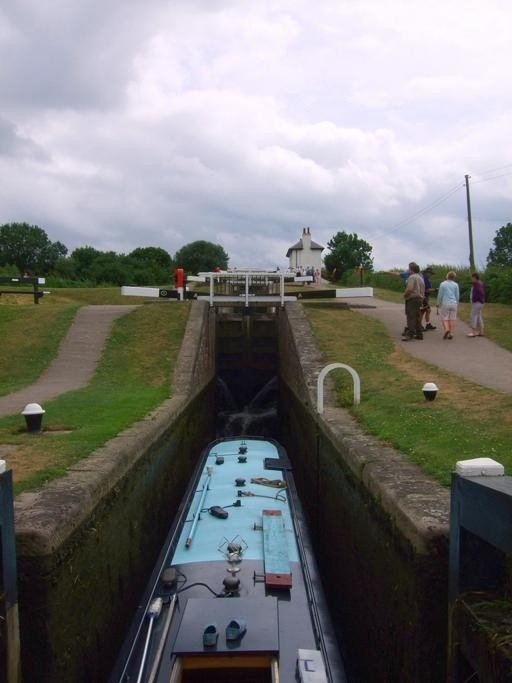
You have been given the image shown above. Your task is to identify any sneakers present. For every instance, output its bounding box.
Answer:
[465,331,485,338]
[442,329,454,340]
[400,321,436,341]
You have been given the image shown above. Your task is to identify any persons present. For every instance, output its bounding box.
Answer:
[464,272,484,336]
[420,267,439,330]
[402,262,426,341]
[289,265,322,285]
[386,264,426,333]
[437,271,460,339]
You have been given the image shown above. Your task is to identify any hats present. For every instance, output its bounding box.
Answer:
[421,267,435,275]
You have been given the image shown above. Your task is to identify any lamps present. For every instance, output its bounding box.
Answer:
[422,382,440,400]
[20,403,46,432]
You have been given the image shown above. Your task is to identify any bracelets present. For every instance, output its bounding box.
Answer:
[434,287,439,291]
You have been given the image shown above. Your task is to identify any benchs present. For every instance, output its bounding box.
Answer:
[1,276,50,304]
